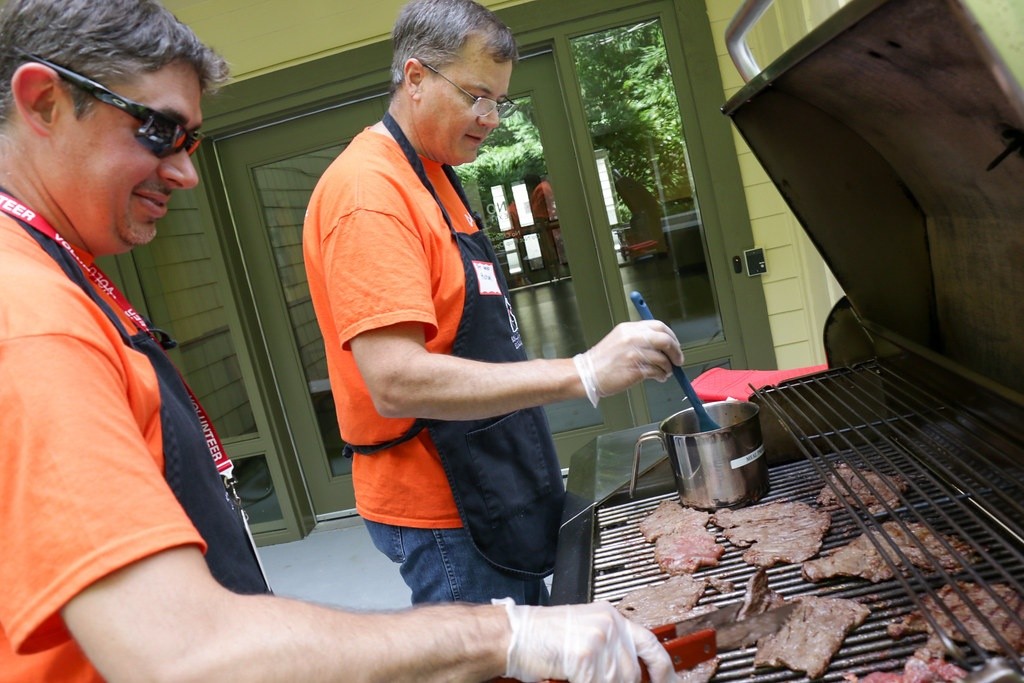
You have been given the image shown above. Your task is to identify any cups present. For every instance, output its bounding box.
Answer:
[628,401,771,514]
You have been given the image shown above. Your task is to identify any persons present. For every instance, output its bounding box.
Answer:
[2,1,674,682]
[303,0,683,611]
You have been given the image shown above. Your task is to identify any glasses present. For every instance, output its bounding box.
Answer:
[420,62,520,118]
[6,45,205,160]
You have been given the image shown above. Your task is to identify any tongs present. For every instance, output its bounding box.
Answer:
[541,591,802,683]
